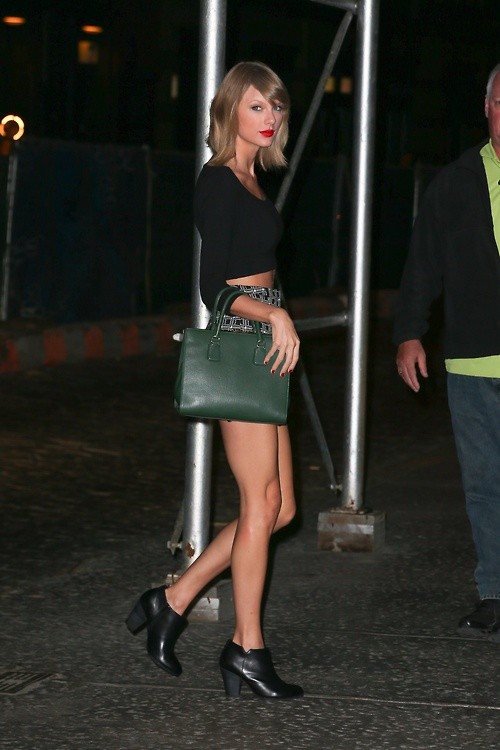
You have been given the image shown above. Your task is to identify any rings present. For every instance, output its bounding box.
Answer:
[399,369,403,375]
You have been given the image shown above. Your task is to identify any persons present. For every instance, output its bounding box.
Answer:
[396,64,500,635]
[125,61,304,701]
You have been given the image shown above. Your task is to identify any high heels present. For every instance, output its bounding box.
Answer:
[125,585,189,677]
[219,640,304,700]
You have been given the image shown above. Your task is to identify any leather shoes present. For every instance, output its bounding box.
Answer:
[459,599,500,633]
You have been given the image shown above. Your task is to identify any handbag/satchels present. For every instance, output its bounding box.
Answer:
[174,284,290,424]
